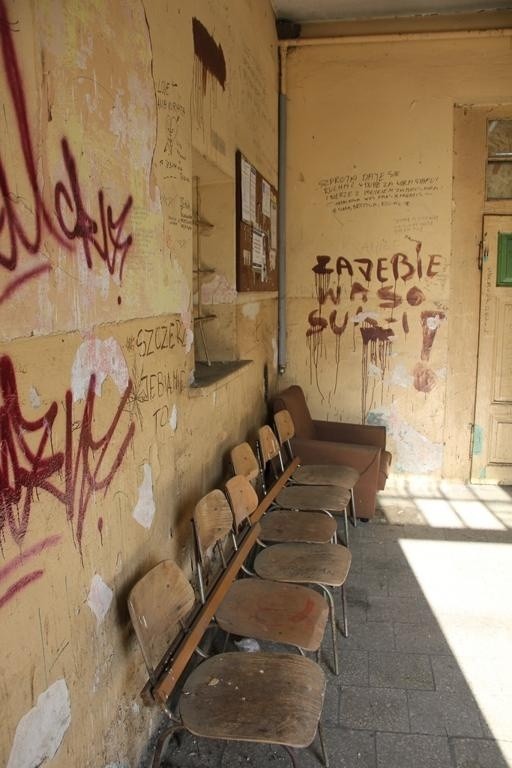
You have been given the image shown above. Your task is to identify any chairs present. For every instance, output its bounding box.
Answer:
[226,409,359,638]
[127,564,339,767]
[183,478,353,702]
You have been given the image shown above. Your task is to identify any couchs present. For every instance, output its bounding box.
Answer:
[268,387,391,530]
